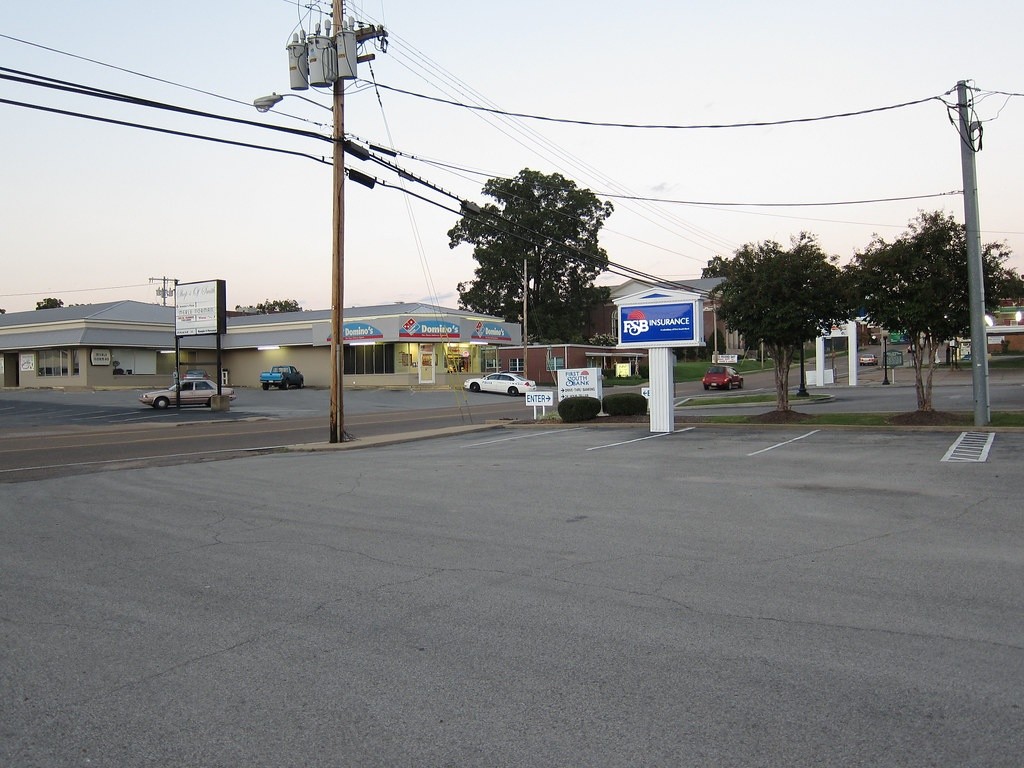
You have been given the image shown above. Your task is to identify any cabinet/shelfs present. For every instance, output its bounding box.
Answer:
[222,372,227,386]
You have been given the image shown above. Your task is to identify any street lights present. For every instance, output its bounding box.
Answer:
[882,330,890,386]
[253,92,358,446]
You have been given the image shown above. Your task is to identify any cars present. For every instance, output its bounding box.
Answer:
[464,370,537,398]
[859,353,878,367]
[908,344,916,352]
[703,365,743,391]
[138,378,236,411]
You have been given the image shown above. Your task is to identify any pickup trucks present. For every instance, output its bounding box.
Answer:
[259,366,304,391]
